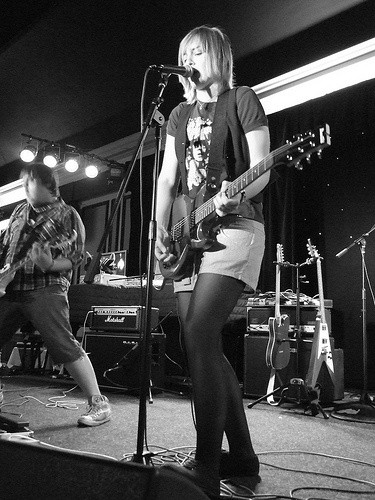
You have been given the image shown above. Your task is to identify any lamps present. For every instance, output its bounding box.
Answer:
[84,158,100,178]
[64,151,81,173]
[42,145,62,167]
[20,135,39,163]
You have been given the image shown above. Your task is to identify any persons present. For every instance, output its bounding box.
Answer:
[154,26,270,495]
[0,163,111,426]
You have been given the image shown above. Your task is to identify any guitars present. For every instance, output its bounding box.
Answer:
[0,227,78,297]
[265,242,291,371]
[159,123,332,281]
[304,241,336,387]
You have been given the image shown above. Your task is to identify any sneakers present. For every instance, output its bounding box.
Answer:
[75,395,113,426]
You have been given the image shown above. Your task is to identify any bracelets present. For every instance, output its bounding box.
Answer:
[240,190,245,203]
[44,261,54,272]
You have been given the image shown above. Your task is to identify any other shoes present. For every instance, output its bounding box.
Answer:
[219,447,259,477]
[184,459,221,496]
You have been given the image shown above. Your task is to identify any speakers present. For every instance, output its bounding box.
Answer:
[243,334,335,404]
[84,333,165,394]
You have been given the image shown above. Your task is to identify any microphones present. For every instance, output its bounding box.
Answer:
[150,64,194,78]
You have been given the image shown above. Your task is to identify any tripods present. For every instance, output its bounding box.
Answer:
[247,228,375,423]
